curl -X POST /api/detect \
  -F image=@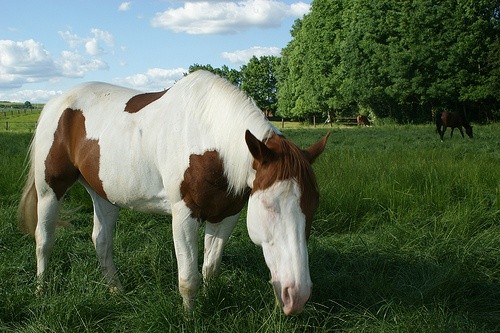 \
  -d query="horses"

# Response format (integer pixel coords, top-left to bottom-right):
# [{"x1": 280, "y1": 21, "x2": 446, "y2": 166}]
[
  {"x1": 16, "y1": 69, "x2": 330, "y2": 323},
  {"x1": 434, "y1": 112, "x2": 472, "y2": 143},
  {"x1": 356, "y1": 115, "x2": 370, "y2": 127}
]
